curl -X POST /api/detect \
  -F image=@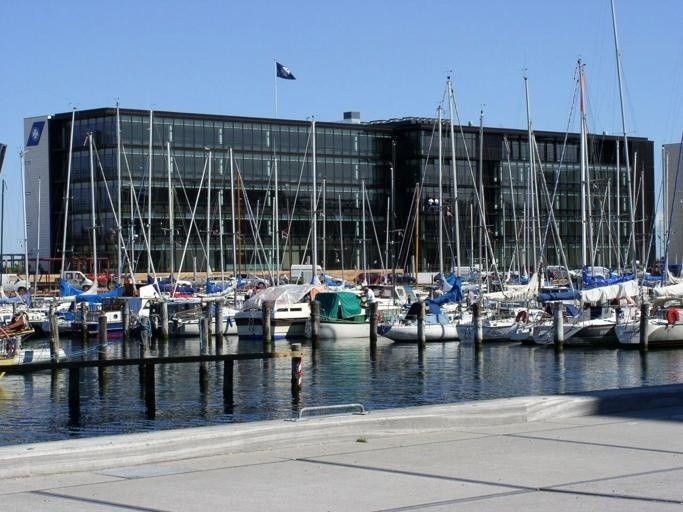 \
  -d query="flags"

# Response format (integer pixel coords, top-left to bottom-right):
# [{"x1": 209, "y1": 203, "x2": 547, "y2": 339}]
[{"x1": 277, "y1": 62, "x2": 296, "y2": 80}]
